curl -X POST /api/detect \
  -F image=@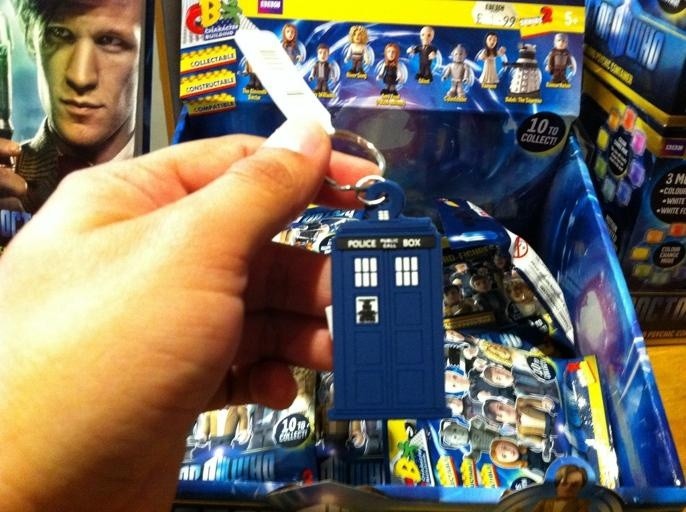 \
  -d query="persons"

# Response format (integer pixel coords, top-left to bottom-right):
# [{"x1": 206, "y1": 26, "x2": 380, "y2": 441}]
[
  {"x1": 280, "y1": 26, "x2": 576, "y2": 107},
  {"x1": 0, "y1": 115, "x2": 386, "y2": 512},
  {"x1": 191, "y1": 405, "x2": 250, "y2": 459},
  {"x1": 0, "y1": 1, "x2": 141, "y2": 248}
]
